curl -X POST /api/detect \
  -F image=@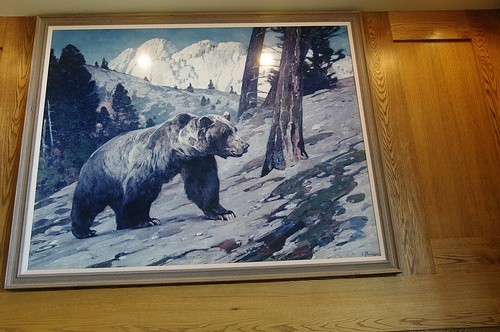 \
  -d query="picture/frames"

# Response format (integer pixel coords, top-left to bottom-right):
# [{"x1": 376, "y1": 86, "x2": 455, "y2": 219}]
[{"x1": 5, "y1": 13, "x2": 402, "y2": 286}]
